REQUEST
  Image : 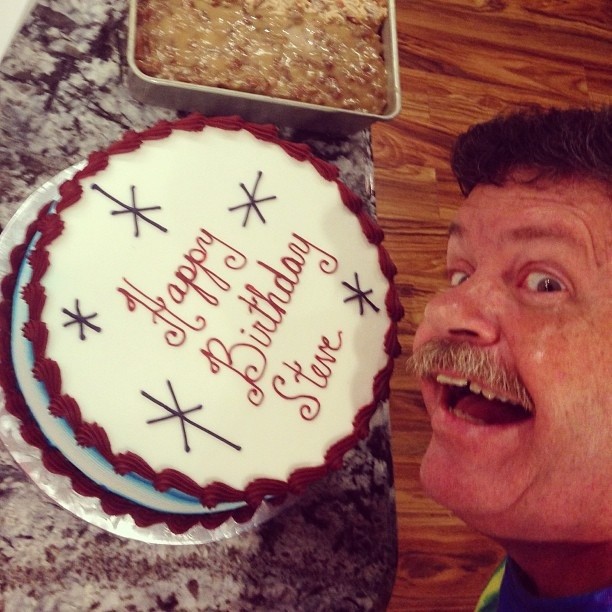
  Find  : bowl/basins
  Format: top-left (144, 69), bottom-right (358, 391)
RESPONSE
top-left (126, 0), bottom-right (403, 140)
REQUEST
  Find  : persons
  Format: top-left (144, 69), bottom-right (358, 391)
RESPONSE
top-left (410, 105), bottom-right (611, 608)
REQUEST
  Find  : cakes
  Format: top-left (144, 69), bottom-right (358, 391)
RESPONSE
top-left (0, 112), bottom-right (405, 547)
